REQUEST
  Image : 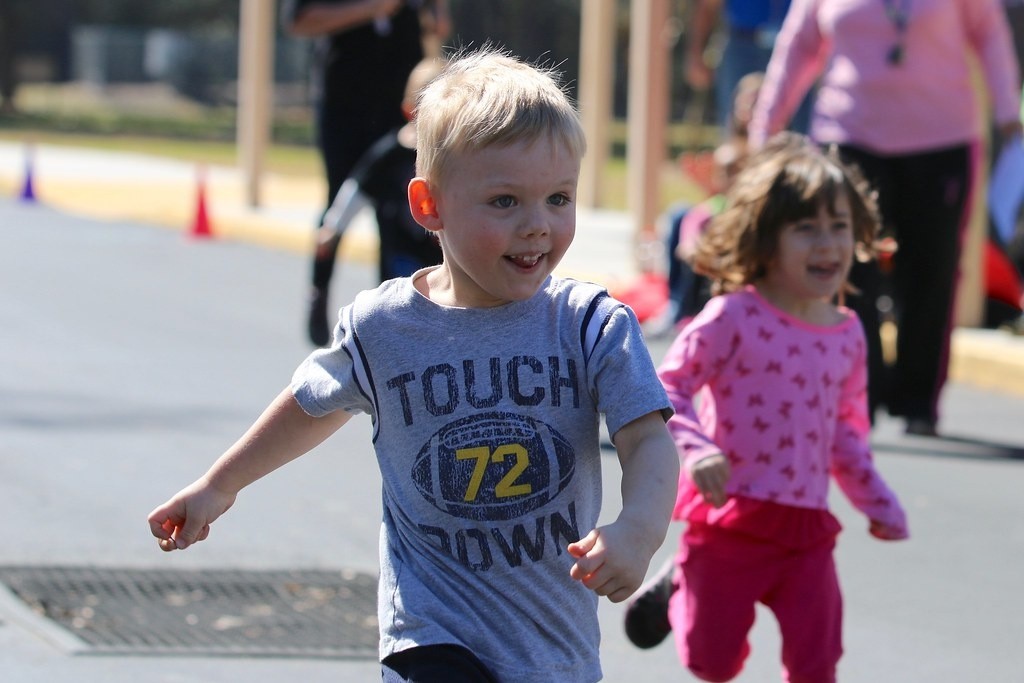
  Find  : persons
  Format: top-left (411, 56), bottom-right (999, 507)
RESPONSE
top-left (288, 1), bottom-right (1024, 683)
top-left (147, 49), bottom-right (680, 683)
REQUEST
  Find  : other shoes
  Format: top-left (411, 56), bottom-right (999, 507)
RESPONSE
top-left (641, 320), bottom-right (674, 339)
top-left (907, 421), bottom-right (936, 435)
top-left (310, 298), bottom-right (330, 344)
top-left (625, 560), bottom-right (680, 650)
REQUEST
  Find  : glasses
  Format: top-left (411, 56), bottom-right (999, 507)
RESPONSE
top-left (888, 9), bottom-right (909, 66)
